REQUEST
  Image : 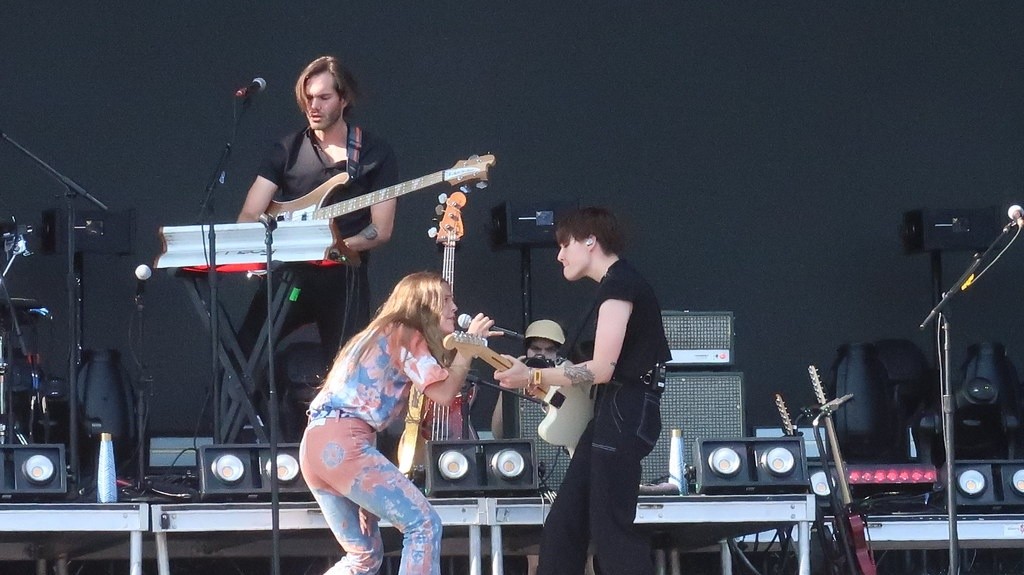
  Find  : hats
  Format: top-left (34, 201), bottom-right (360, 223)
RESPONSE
top-left (525, 320), bottom-right (566, 344)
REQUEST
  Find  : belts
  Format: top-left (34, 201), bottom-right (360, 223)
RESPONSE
top-left (311, 408), bottom-right (359, 419)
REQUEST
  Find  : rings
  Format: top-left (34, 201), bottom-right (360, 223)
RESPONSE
top-left (488, 331), bottom-right (491, 336)
top-left (482, 319), bottom-right (485, 324)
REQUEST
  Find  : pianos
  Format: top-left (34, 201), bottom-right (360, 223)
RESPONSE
top-left (152, 218), bottom-right (361, 443)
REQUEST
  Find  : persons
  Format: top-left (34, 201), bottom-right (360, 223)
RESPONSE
top-left (220, 56), bottom-right (400, 502)
top-left (491, 319), bottom-right (596, 575)
top-left (298, 273), bottom-right (504, 575)
top-left (492, 208), bottom-right (672, 575)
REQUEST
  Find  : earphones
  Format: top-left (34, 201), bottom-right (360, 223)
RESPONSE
top-left (586, 238), bottom-right (593, 246)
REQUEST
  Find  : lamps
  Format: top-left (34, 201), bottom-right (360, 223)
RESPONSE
top-left (76, 347), bottom-right (139, 473)
top-left (958, 342), bottom-right (1023, 459)
top-left (827, 338), bottom-right (901, 460)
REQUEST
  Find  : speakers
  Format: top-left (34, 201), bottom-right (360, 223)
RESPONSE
top-left (512, 371), bottom-right (746, 498)
top-left (662, 309), bottom-right (736, 369)
top-left (904, 203), bottom-right (1007, 255)
top-left (491, 198), bottom-right (581, 249)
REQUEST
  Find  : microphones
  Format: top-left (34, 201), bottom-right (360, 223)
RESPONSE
top-left (458, 314), bottom-right (529, 340)
top-left (236, 78), bottom-right (266, 96)
top-left (134, 264), bottom-right (152, 297)
top-left (1008, 204), bottom-right (1024, 228)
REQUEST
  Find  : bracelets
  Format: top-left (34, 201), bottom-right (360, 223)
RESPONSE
top-left (527, 366), bottom-right (541, 392)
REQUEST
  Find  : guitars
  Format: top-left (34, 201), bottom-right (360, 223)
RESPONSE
top-left (773, 391), bottom-right (844, 575)
top-left (396, 191), bottom-right (477, 479)
top-left (808, 364), bottom-right (877, 575)
top-left (246, 153), bottom-right (496, 276)
top-left (443, 332), bottom-right (596, 458)
